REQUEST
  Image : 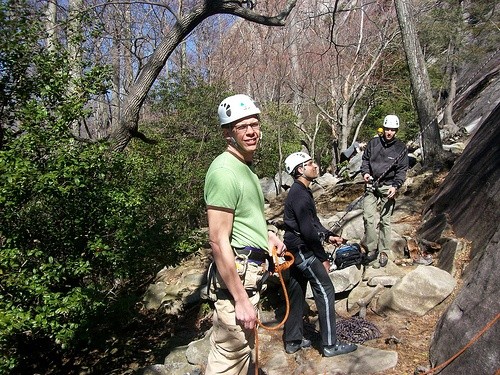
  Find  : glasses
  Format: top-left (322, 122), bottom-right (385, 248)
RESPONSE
top-left (232, 124), bottom-right (258, 133)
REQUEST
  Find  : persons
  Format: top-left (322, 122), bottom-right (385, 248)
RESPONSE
top-left (203, 94), bottom-right (287, 375)
top-left (374, 128), bottom-right (384, 138)
top-left (360, 115), bottom-right (409, 266)
top-left (283, 152), bottom-right (359, 356)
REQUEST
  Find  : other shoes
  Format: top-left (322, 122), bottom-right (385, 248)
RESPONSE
top-left (379, 252), bottom-right (388, 266)
top-left (323, 340), bottom-right (358, 356)
top-left (285, 339), bottom-right (311, 354)
top-left (367, 251), bottom-right (376, 260)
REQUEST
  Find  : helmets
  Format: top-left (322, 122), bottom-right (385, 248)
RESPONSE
top-left (284, 151), bottom-right (313, 173)
top-left (216, 94), bottom-right (261, 125)
top-left (378, 128), bottom-right (383, 133)
top-left (382, 114), bottom-right (399, 128)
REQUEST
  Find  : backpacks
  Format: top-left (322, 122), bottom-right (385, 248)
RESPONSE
top-left (334, 243), bottom-right (363, 270)
